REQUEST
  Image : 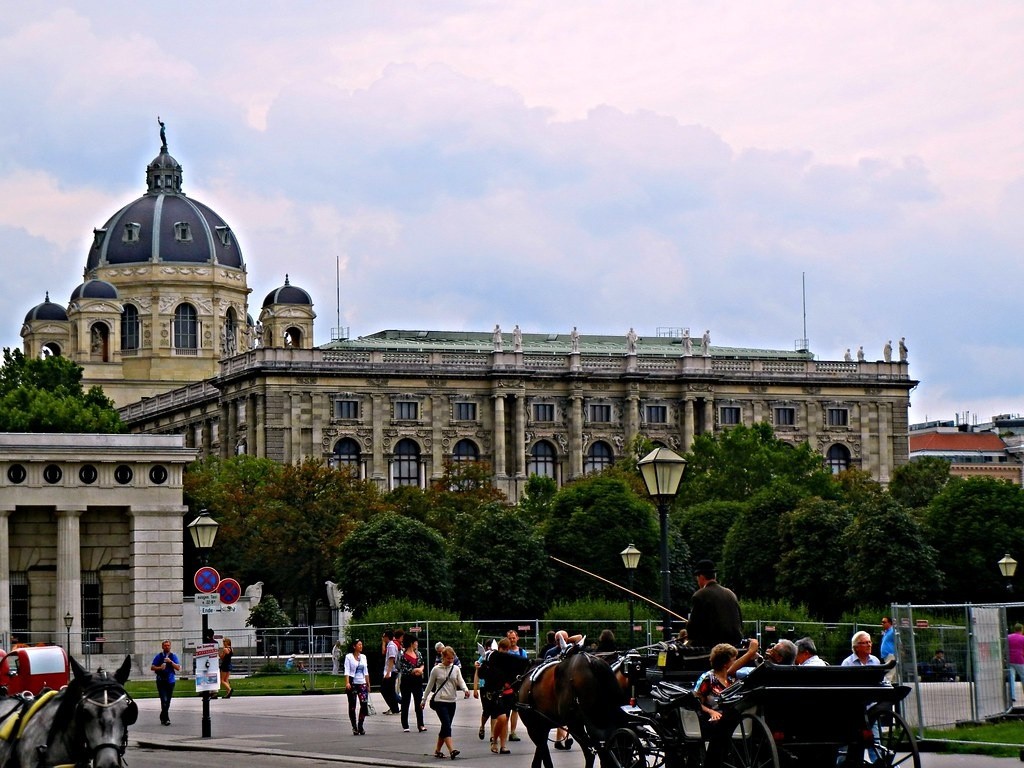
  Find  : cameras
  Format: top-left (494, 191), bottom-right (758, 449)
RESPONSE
top-left (741, 638), bottom-right (760, 648)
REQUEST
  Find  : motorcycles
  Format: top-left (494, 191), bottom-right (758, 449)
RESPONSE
top-left (0, 645), bottom-right (70, 696)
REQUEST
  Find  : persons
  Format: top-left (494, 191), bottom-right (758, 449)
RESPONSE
top-left (1007, 623), bottom-right (1024, 701)
top-left (626, 327), bottom-right (637, 354)
top-left (473, 630), bottom-right (618, 754)
top-left (702, 330), bottom-right (711, 355)
top-left (243, 321), bottom-right (264, 349)
top-left (380, 628), bottom-right (462, 733)
top-left (857, 346), bottom-right (864, 361)
top-left (682, 329), bottom-right (693, 355)
top-left (222, 330), bottom-right (234, 357)
top-left (513, 325), bottom-right (522, 351)
top-left (898, 337), bottom-right (908, 361)
top-left (493, 325), bottom-right (502, 350)
top-left (344, 639), bottom-right (371, 736)
top-left (844, 349), bottom-right (852, 361)
top-left (158, 117), bottom-right (166, 147)
top-left (284, 333), bottom-right (291, 348)
top-left (151, 640), bottom-right (181, 726)
top-left (678, 560), bottom-right (746, 645)
top-left (571, 327), bottom-right (579, 352)
top-left (92, 332), bottom-right (103, 354)
top-left (692, 614), bottom-right (905, 763)
top-left (883, 341), bottom-right (892, 362)
top-left (332, 640), bottom-right (342, 674)
top-left (286, 654), bottom-right (300, 670)
top-left (420, 646), bottom-right (470, 760)
top-left (207, 628), bottom-right (233, 699)
top-left (930, 649), bottom-right (956, 682)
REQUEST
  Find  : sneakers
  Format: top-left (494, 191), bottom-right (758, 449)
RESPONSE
top-left (383, 709), bottom-right (393, 715)
top-left (161, 719), bottom-right (170, 724)
top-left (164, 719), bottom-right (171, 726)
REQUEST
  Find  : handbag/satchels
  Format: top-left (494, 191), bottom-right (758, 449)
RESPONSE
top-left (430, 699), bottom-right (435, 710)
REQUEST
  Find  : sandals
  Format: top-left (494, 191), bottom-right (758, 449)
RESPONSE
top-left (450, 750), bottom-right (460, 760)
top-left (434, 750), bottom-right (446, 758)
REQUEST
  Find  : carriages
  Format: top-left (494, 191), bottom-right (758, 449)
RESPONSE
top-left (477, 627), bottom-right (919, 768)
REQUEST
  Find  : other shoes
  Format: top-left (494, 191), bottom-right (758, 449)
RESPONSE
top-left (500, 747), bottom-right (510, 755)
top-left (479, 727), bottom-right (485, 740)
top-left (353, 728), bottom-right (359, 736)
top-left (554, 741), bottom-right (566, 750)
top-left (359, 727), bottom-right (365, 735)
top-left (509, 733), bottom-right (521, 742)
top-left (404, 728), bottom-right (409, 732)
top-left (491, 742), bottom-right (498, 754)
top-left (566, 738), bottom-right (573, 750)
top-left (418, 726), bottom-right (427, 733)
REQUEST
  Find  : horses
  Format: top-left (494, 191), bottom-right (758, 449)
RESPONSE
top-left (0, 654), bottom-right (139, 768)
top-left (475, 641), bottom-right (630, 768)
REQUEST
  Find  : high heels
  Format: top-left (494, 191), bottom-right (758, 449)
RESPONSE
top-left (222, 688), bottom-right (234, 699)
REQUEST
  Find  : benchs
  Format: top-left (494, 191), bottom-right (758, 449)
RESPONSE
top-left (659, 646), bottom-right (755, 680)
top-left (728, 661), bottom-right (898, 732)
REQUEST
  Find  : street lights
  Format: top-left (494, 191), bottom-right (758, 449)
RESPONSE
top-left (997, 553), bottom-right (1018, 625)
top-left (636, 439), bottom-right (689, 648)
top-left (187, 507), bottom-right (222, 738)
top-left (63, 611), bottom-right (74, 656)
top-left (620, 541), bottom-right (643, 650)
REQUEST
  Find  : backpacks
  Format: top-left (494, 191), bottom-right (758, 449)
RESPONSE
top-left (395, 650), bottom-right (404, 671)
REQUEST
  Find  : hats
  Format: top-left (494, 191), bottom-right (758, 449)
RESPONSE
top-left (694, 560), bottom-right (720, 574)
